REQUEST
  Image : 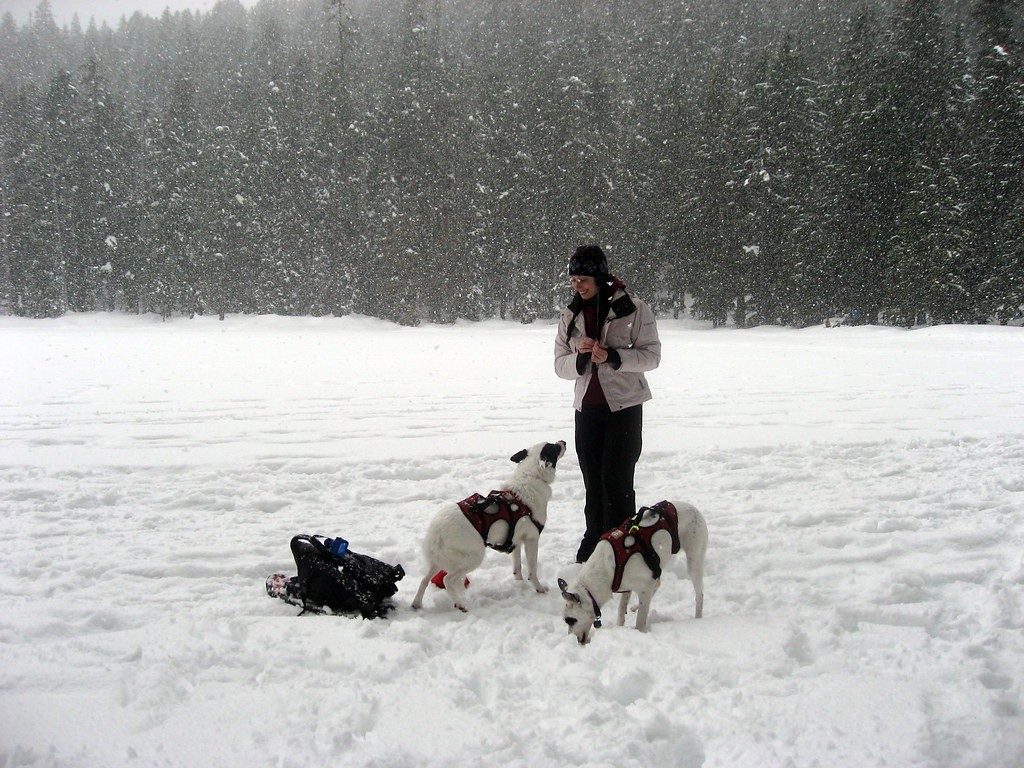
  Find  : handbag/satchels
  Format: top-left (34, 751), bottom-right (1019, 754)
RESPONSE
top-left (290, 535), bottom-right (406, 615)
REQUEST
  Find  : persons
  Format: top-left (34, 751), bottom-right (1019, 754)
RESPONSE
top-left (555, 245), bottom-right (661, 564)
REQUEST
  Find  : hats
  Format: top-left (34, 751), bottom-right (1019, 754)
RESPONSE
top-left (569, 245), bottom-right (608, 288)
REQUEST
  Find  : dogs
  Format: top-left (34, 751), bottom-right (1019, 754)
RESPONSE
top-left (411, 440), bottom-right (567, 613)
top-left (556, 501), bottom-right (709, 645)
